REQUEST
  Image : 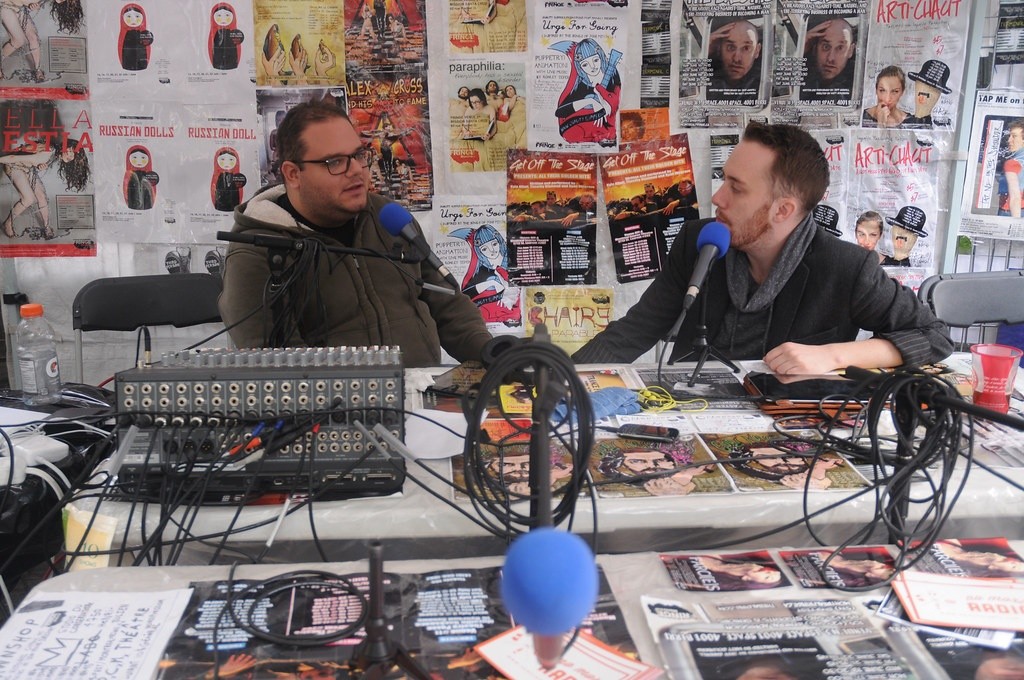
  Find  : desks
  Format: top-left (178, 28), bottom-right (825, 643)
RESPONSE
top-left (2, 363), bottom-right (1024, 680)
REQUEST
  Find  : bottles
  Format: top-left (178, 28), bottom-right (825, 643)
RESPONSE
top-left (15, 304), bottom-right (62, 407)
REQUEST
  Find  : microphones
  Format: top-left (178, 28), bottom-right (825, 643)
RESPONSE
top-left (682, 221), bottom-right (731, 311)
top-left (498, 323), bottom-right (601, 636)
top-left (377, 203), bottom-right (458, 289)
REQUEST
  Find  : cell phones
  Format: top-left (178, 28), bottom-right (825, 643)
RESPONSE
top-left (617, 423), bottom-right (679, 443)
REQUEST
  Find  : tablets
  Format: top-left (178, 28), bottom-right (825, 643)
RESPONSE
top-left (747, 372), bottom-right (893, 406)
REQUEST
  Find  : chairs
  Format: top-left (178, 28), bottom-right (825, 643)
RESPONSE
top-left (72, 274), bottom-right (234, 384)
top-left (917, 269), bottom-right (1024, 330)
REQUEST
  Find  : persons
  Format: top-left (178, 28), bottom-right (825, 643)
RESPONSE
top-left (180, 647), bottom-right (509, 680)
top-left (947, 647), bottom-right (1024, 680)
top-left (508, 190), bottom-right (596, 226)
top-left (799, 17), bottom-right (856, 107)
top-left (360, 123), bottom-right (414, 193)
top-left (997, 119), bottom-right (1024, 219)
top-left (714, 655), bottom-right (806, 680)
top-left (344, 0), bottom-right (409, 65)
top-left (0, 0), bottom-right (84, 80)
top-left (606, 179), bottom-right (696, 221)
top-left (811, 551), bottom-right (898, 588)
top-left (219, 95), bottom-right (493, 369)
top-left (706, 19), bottom-right (762, 107)
top-left (855, 211), bottom-right (892, 265)
top-left (698, 554), bottom-right (784, 592)
top-left (569, 121), bottom-right (955, 375)
top-left (449, 80), bottom-right (527, 173)
top-left (449, 0), bottom-right (528, 54)
top-left (621, 113), bottom-right (644, 142)
top-left (935, 538), bottom-right (1024, 579)
top-left (0, 139), bottom-right (91, 238)
top-left (861, 65), bottom-right (912, 129)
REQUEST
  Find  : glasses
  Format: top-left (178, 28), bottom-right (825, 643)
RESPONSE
top-left (577, 200), bottom-right (593, 207)
top-left (292, 145), bottom-right (375, 175)
top-left (469, 100), bottom-right (481, 105)
top-left (677, 185), bottom-right (694, 192)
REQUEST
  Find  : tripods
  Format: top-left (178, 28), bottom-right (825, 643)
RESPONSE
top-left (666, 257), bottom-right (740, 388)
top-left (348, 544), bottom-right (433, 680)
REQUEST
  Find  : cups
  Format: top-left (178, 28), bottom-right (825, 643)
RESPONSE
top-left (969, 344), bottom-right (1024, 415)
